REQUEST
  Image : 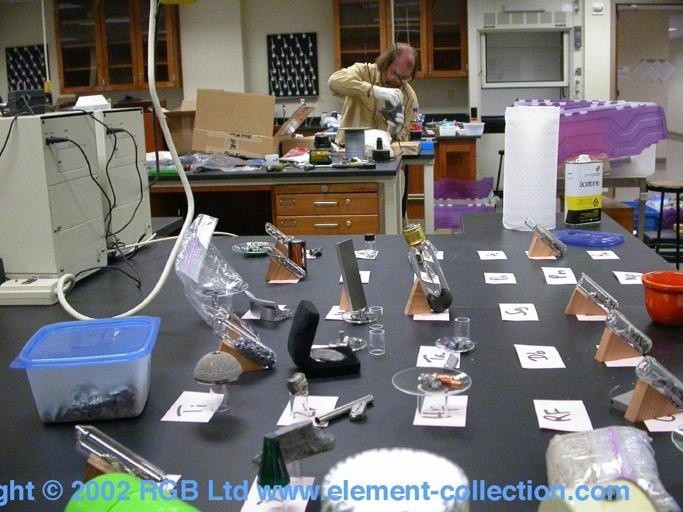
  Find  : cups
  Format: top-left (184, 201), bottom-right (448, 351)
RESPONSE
top-left (453, 317), bottom-right (470, 342)
top-left (365, 305), bottom-right (386, 356)
top-left (372, 149), bottom-right (390, 162)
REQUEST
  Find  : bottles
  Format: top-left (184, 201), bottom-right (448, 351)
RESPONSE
top-left (402, 220), bottom-right (453, 314)
top-left (74, 423), bottom-right (164, 484)
top-left (267, 248), bottom-right (307, 279)
top-left (409, 106), bottom-right (423, 141)
top-left (364, 232), bottom-right (376, 258)
top-left (523, 216), bottom-right (683, 457)
top-left (211, 321), bottom-right (277, 370)
top-left (256, 433), bottom-right (290, 488)
top-left (264, 222), bottom-right (290, 248)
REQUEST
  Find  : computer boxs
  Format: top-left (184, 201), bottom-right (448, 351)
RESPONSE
top-left (2, 113), bottom-right (109, 287)
top-left (95, 105), bottom-right (152, 259)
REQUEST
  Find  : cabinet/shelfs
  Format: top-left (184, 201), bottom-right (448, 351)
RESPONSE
top-left (558, 176), bottom-right (646, 242)
top-left (111, 99), bottom-right (167, 151)
top-left (407, 138), bottom-right (476, 221)
top-left (332, 0), bottom-right (468, 79)
top-left (51, 0), bottom-right (182, 94)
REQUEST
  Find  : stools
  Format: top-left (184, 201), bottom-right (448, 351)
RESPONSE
top-left (643, 178), bottom-right (683, 271)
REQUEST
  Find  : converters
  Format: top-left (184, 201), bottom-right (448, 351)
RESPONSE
top-left (9, 89), bottom-right (46, 114)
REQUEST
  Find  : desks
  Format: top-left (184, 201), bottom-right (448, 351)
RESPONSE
top-left (559, 192), bottom-right (633, 235)
top-left (0, 215), bottom-right (683, 512)
top-left (146, 151), bottom-right (404, 234)
top-left (400, 157), bottom-right (434, 233)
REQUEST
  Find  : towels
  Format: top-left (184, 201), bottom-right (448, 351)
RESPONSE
top-left (516, 96), bottom-right (669, 159)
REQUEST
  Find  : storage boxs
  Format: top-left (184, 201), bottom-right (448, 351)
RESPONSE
top-left (191, 88), bottom-right (315, 159)
top-left (11, 315), bottom-right (163, 422)
top-left (147, 104), bottom-right (195, 156)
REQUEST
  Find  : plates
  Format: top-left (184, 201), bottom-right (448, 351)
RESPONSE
top-left (340, 310), bottom-right (376, 324)
top-left (231, 242), bottom-right (271, 255)
top-left (327, 337), bottom-right (368, 352)
top-left (391, 366), bottom-right (473, 398)
top-left (434, 336), bottom-right (475, 353)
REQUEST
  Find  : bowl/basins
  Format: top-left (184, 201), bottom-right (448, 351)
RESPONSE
top-left (455, 121), bottom-right (486, 136)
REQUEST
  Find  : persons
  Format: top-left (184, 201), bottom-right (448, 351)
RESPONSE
top-left (328, 43), bottom-right (419, 230)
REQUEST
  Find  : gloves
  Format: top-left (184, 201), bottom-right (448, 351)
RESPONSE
top-left (385, 112), bottom-right (405, 136)
top-left (369, 85), bottom-right (404, 109)
